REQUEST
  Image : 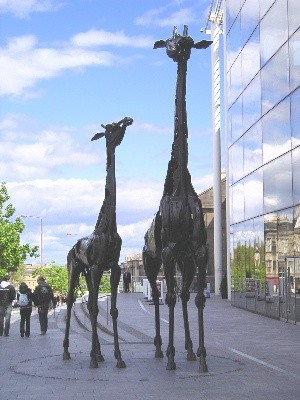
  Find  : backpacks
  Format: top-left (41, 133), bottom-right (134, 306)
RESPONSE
top-left (18, 290), bottom-right (30, 306)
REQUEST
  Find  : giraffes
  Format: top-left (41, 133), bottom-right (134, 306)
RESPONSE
top-left (62, 117), bottom-right (134, 369)
top-left (142, 24), bottom-right (214, 373)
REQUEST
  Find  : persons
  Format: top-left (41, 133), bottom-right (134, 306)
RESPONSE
top-left (123, 268), bottom-right (132, 293)
top-left (0, 274), bottom-right (16, 337)
top-left (16, 282), bottom-right (33, 338)
top-left (33, 276), bottom-right (57, 335)
